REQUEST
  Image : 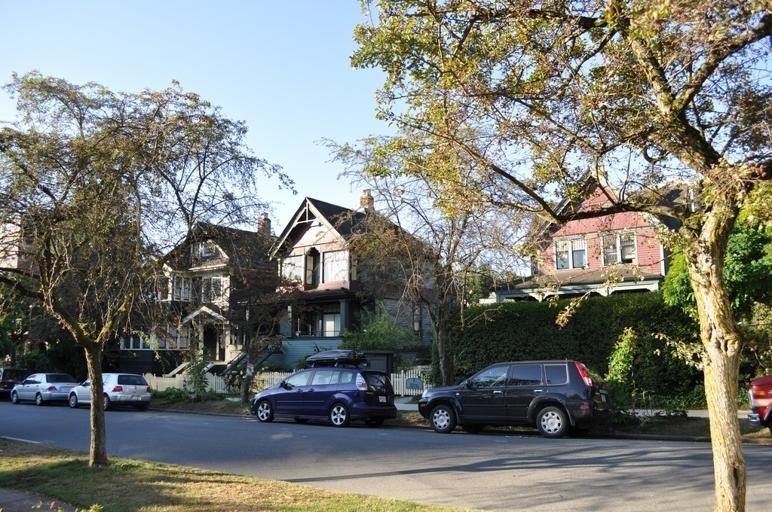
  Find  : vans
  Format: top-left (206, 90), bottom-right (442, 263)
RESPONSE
top-left (249, 349), bottom-right (397, 428)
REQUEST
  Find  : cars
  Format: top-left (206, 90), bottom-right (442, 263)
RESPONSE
top-left (418, 358), bottom-right (612, 438)
top-left (747, 374), bottom-right (772, 436)
top-left (0, 367), bottom-right (153, 411)
